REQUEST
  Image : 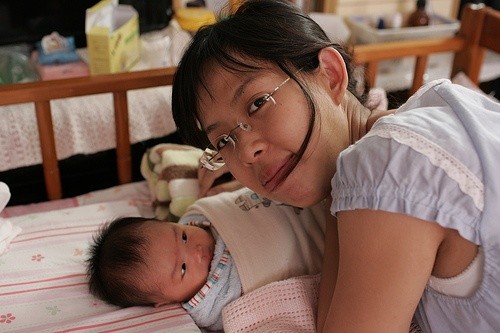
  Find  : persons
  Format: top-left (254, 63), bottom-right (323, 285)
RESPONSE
top-left (172, 0), bottom-right (500, 333)
top-left (87, 186), bottom-right (326, 331)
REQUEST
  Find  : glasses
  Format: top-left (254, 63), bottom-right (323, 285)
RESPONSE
top-left (199, 68), bottom-right (304, 170)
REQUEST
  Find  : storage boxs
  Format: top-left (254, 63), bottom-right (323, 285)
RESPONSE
top-left (85, 0), bottom-right (141, 75)
top-left (348, 12), bottom-right (461, 74)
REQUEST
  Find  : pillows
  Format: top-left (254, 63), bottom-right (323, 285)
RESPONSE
top-left (0, 198), bottom-right (202, 333)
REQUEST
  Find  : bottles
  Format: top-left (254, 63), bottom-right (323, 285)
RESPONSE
top-left (406, 0), bottom-right (429, 27)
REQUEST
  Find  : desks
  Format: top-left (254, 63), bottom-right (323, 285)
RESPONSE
top-left (0, 13), bottom-right (350, 207)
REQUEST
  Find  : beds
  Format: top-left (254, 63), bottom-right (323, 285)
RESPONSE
top-left (0, 4), bottom-right (500, 333)
